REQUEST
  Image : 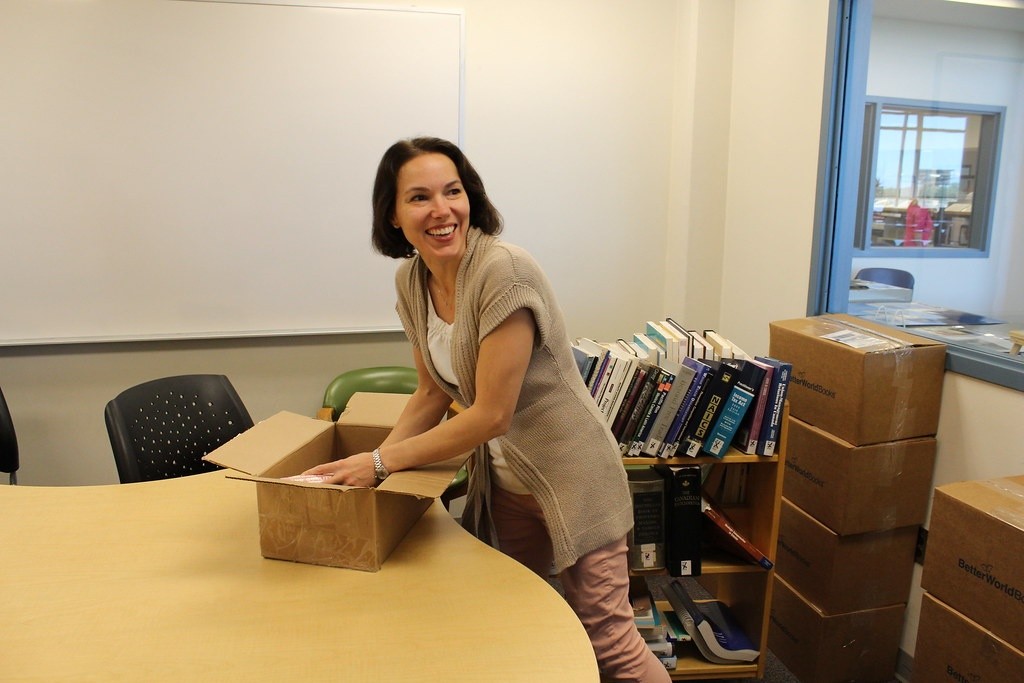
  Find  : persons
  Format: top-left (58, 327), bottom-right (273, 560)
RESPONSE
top-left (904, 185), bottom-right (934, 247)
top-left (300, 138), bottom-right (677, 683)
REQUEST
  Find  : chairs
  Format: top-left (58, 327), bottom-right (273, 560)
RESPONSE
top-left (854, 268), bottom-right (915, 290)
top-left (316, 367), bottom-right (470, 513)
top-left (104, 373), bottom-right (255, 485)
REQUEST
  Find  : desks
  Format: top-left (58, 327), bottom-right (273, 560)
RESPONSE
top-left (0, 469), bottom-right (602, 683)
top-left (848, 279), bottom-right (1024, 359)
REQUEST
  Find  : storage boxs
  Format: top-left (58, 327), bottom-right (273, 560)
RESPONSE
top-left (766, 572), bottom-right (907, 683)
top-left (769, 313), bottom-right (949, 447)
top-left (921, 475), bottom-right (1024, 653)
top-left (201, 391), bottom-right (475, 573)
top-left (781, 414), bottom-right (937, 537)
top-left (774, 496), bottom-right (917, 616)
top-left (910, 590), bottom-right (1024, 683)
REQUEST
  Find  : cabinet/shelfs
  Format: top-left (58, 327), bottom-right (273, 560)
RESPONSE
top-left (621, 400), bottom-right (790, 683)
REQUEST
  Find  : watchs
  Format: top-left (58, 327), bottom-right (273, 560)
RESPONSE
top-left (371, 449), bottom-right (389, 480)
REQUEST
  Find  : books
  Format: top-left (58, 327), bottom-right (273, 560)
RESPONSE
top-left (616, 574), bottom-right (760, 670)
top-left (623, 460), bottom-right (774, 576)
top-left (567, 319), bottom-right (793, 461)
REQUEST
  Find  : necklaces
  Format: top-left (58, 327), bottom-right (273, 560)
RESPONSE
top-left (436, 282), bottom-right (455, 314)
